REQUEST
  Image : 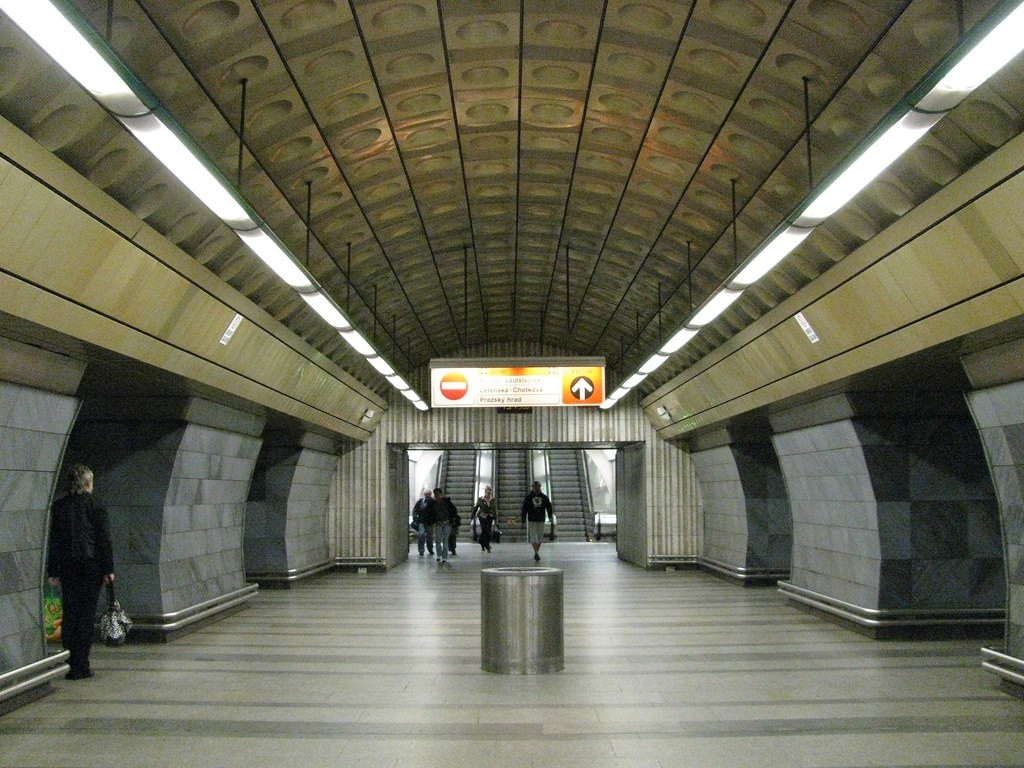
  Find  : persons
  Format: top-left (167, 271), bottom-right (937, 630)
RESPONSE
top-left (431, 488), bottom-right (458, 562)
top-left (48, 463), bottom-right (114, 680)
top-left (521, 481), bottom-right (553, 561)
top-left (413, 488), bottom-right (436, 556)
top-left (470, 484), bottom-right (499, 553)
top-left (448, 515), bottom-right (461, 555)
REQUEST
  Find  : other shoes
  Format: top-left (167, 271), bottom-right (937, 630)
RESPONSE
top-left (482, 546), bottom-right (485, 551)
top-left (65, 660), bottom-right (95, 679)
top-left (419, 553), bottom-right (423, 557)
top-left (534, 553), bottom-right (540, 561)
top-left (429, 551), bottom-right (434, 554)
top-left (437, 556), bottom-right (440, 561)
top-left (451, 550), bottom-right (456, 555)
top-left (487, 549), bottom-right (491, 553)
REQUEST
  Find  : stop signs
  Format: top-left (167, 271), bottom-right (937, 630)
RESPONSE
top-left (440, 372), bottom-right (468, 400)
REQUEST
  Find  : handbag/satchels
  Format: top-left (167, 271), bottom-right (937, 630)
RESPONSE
top-left (411, 520), bottom-right (419, 531)
top-left (102, 576), bottom-right (133, 647)
top-left (44, 582), bottom-right (62, 640)
top-left (493, 530), bottom-right (500, 543)
